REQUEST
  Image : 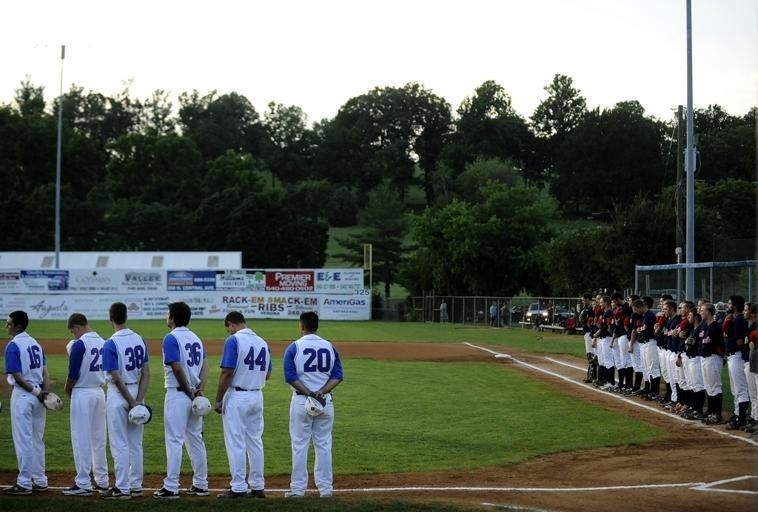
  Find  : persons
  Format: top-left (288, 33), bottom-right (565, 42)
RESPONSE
top-left (214, 310), bottom-right (272, 498)
top-left (439, 298), bottom-right (580, 334)
top-left (152, 300), bottom-right (211, 496)
top-left (580, 289), bottom-right (758, 436)
top-left (101, 303), bottom-right (150, 499)
top-left (283, 311), bottom-right (344, 498)
top-left (2, 311), bottom-right (49, 497)
top-left (59, 312), bottom-right (111, 498)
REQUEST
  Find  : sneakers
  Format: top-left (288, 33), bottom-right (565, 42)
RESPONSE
top-left (724, 415), bottom-right (757, 438)
top-left (284, 492), bottom-right (302, 498)
top-left (185, 485), bottom-right (209, 496)
top-left (217, 489), bottom-right (247, 499)
top-left (583, 377), bottom-right (617, 391)
top-left (250, 489), bottom-right (266, 499)
top-left (62, 483), bottom-right (143, 500)
top-left (153, 487), bottom-right (179, 500)
top-left (657, 398), bottom-right (722, 425)
top-left (31, 481), bottom-right (48, 492)
top-left (2, 484), bottom-right (32, 495)
top-left (610, 386), bottom-right (661, 401)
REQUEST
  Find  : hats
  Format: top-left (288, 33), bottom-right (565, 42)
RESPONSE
top-left (306, 391), bottom-right (326, 417)
top-left (192, 396), bottom-right (211, 417)
top-left (44, 392), bottom-right (63, 411)
top-left (128, 403), bottom-right (152, 426)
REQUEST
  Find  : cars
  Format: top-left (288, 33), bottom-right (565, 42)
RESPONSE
top-left (551, 305), bottom-right (574, 324)
top-left (47, 275), bottom-right (66, 291)
top-left (511, 305), bottom-right (523, 320)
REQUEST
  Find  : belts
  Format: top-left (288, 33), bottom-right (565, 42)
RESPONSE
top-left (236, 386), bottom-right (247, 391)
top-left (296, 391), bottom-right (304, 395)
top-left (176, 387), bottom-right (184, 392)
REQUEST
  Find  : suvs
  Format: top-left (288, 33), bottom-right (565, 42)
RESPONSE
top-left (524, 303), bottom-right (550, 325)
